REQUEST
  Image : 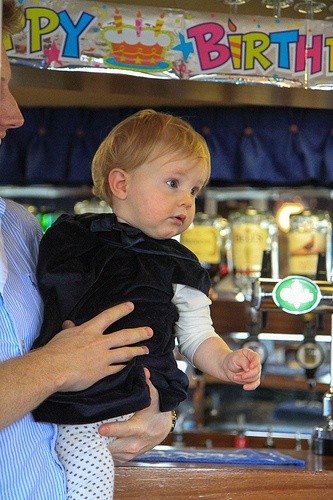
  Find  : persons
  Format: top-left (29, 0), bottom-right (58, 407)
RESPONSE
top-left (1, 1), bottom-right (176, 499)
top-left (28, 110), bottom-right (263, 499)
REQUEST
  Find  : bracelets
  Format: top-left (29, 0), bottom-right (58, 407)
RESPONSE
top-left (171, 410), bottom-right (176, 432)
